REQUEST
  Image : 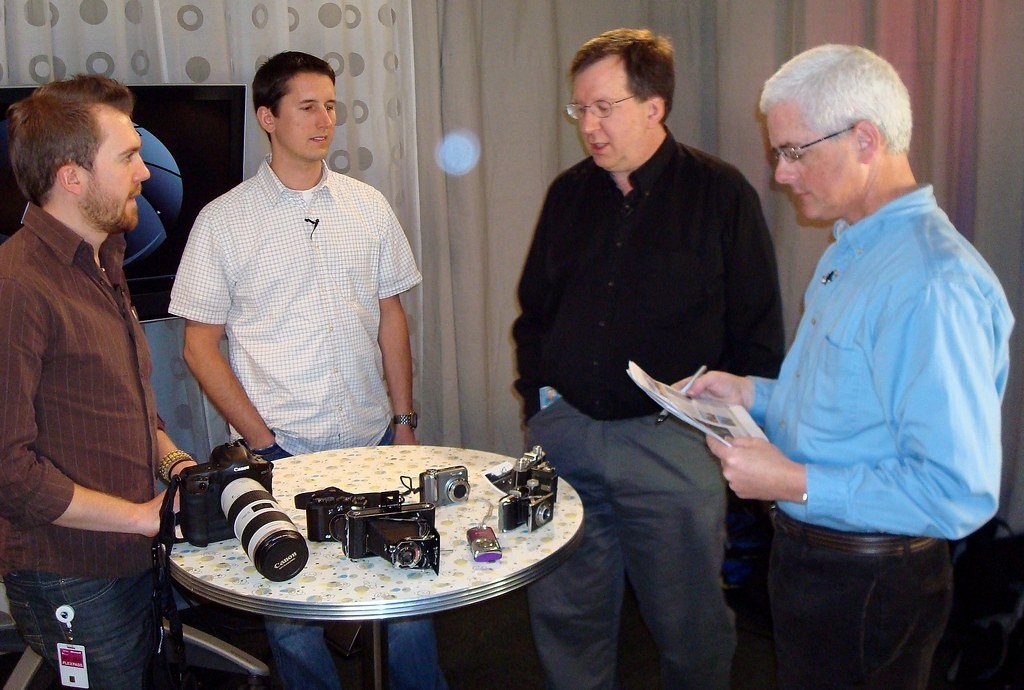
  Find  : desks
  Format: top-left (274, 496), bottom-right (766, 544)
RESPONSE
top-left (170, 445), bottom-right (585, 690)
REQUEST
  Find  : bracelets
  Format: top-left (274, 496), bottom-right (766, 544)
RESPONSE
top-left (157, 449), bottom-right (193, 482)
top-left (799, 492), bottom-right (808, 506)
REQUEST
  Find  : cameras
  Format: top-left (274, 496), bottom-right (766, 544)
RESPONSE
top-left (498, 488), bottom-right (555, 533)
top-left (305, 490), bottom-right (399, 543)
top-left (419, 466), bottom-right (471, 507)
top-left (344, 502), bottom-right (438, 570)
top-left (175, 441), bottom-right (310, 582)
top-left (513, 444), bottom-right (559, 500)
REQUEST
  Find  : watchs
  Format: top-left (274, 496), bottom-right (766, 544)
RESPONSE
top-left (392, 412), bottom-right (417, 429)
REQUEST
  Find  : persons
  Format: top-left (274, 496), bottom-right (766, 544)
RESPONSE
top-left (166, 51), bottom-right (450, 690)
top-left (0, 74), bottom-right (199, 690)
top-left (511, 27), bottom-right (786, 690)
top-left (671, 43), bottom-right (1016, 690)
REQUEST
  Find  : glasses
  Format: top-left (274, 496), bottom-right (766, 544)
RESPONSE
top-left (770, 124), bottom-right (856, 165)
top-left (566, 93), bottom-right (640, 120)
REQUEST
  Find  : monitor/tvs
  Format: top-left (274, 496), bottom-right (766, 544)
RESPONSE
top-left (1, 84), bottom-right (247, 324)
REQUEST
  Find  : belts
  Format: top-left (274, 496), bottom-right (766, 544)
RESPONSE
top-left (769, 502), bottom-right (938, 556)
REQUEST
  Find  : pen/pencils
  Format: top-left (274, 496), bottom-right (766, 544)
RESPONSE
top-left (656, 364), bottom-right (706, 424)
